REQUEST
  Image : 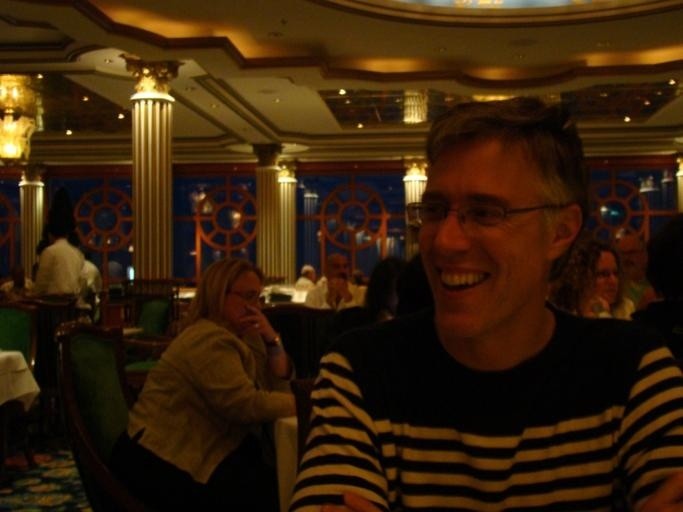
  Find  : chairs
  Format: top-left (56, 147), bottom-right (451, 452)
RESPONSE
top-left (0, 277), bottom-right (336, 511)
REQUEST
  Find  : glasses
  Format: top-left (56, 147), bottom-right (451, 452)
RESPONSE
top-left (227, 292), bottom-right (265, 306)
top-left (408, 202), bottom-right (557, 229)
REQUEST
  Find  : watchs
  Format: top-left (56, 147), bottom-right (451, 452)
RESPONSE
top-left (264, 331), bottom-right (280, 348)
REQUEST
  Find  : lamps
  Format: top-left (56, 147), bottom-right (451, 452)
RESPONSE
top-left (0, 72), bottom-right (40, 167)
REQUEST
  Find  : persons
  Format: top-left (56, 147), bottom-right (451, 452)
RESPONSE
top-left (125, 257), bottom-right (297, 512)
top-left (1, 218), bottom-right (104, 385)
top-left (550, 213), bottom-right (683, 369)
top-left (287, 104), bottom-right (683, 511)
top-left (289, 251), bottom-right (436, 380)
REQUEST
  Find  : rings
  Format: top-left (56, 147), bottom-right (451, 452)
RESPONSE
top-left (255, 323), bottom-right (261, 329)
